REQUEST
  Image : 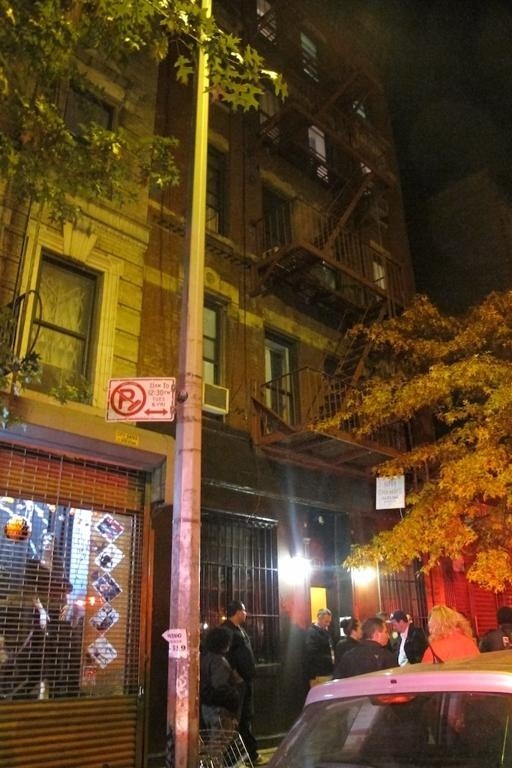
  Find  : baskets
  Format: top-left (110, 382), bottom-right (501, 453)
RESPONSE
top-left (199, 729), bottom-right (254, 768)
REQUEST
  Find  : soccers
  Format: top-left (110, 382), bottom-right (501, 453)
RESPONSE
top-left (4, 515), bottom-right (33, 541)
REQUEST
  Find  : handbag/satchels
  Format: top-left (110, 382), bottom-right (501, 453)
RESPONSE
top-left (201, 675), bottom-right (242, 712)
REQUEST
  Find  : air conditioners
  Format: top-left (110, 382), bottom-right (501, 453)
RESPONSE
top-left (203, 382), bottom-right (230, 417)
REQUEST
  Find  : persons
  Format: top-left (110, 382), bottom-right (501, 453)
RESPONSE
top-left (219, 600), bottom-right (271, 766)
top-left (304, 603), bottom-right (512, 733)
top-left (199, 627), bottom-right (244, 757)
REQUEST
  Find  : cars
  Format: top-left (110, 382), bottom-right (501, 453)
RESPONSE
top-left (269, 647), bottom-right (512, 768)
